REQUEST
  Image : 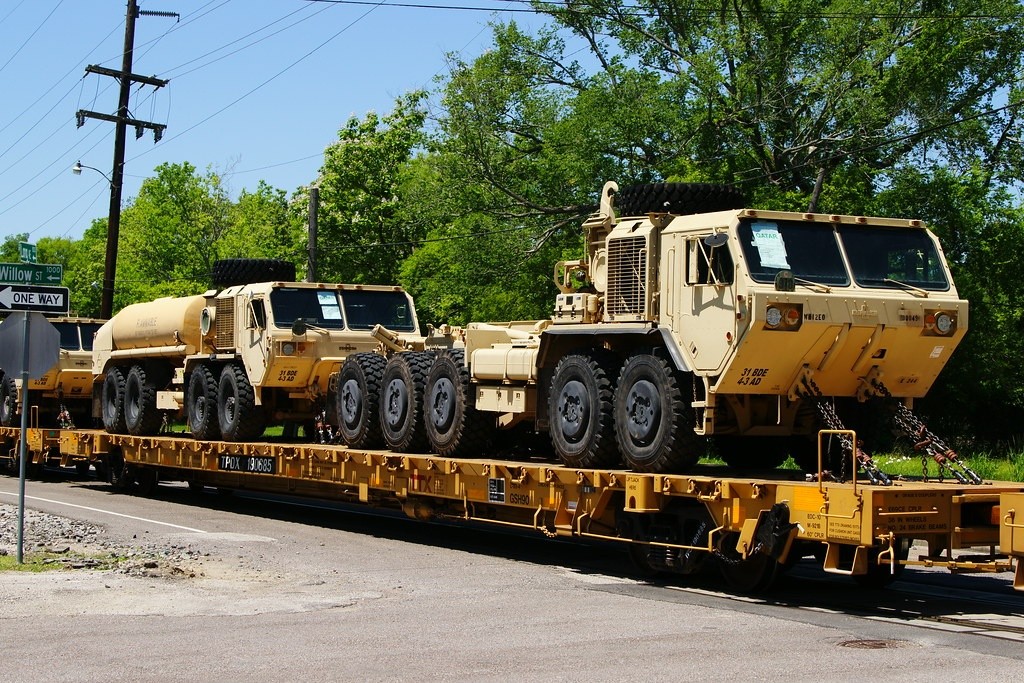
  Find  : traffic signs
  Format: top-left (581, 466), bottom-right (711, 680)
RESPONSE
top-left (2, 286), bottom-right (68, 308)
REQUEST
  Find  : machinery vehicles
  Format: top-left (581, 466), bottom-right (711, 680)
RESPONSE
top-left (333, 183), bottom-right (971, 468)
top-left (0, 281), bottom-right (426, 441)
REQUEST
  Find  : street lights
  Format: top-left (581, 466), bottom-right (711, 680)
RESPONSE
top-left (74, 157), bottom-right (125, 320)
